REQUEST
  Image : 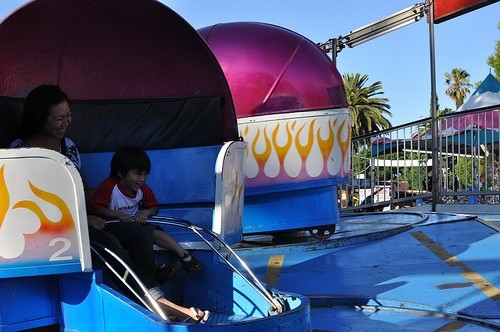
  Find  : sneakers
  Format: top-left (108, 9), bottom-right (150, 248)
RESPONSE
top-left (181, 253), bottom-right (203, 273)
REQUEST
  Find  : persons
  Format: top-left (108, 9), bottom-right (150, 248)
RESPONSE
top-left (8, 83), bottom-right (211, 325)
top-left (87, 145), bottom-right (204, 289)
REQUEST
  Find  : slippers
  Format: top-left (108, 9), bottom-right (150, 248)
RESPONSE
top-left (182, 307), bottom-right (210, 324)
